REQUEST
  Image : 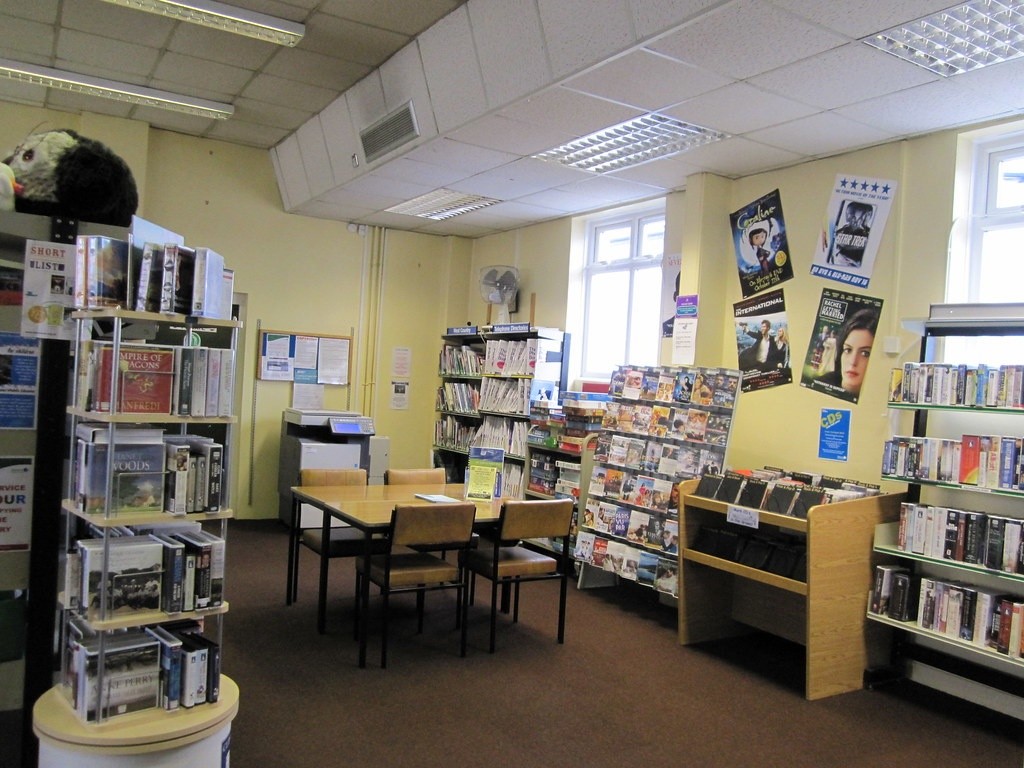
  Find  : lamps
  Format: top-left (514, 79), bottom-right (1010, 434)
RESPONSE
top-left (0, 58), bottom-right (236, 122)
top-left (104, 0), bottom-right (307, 48)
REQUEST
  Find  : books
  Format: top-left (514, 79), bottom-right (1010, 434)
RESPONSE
top-left (826, 200), bottom-right (879, 268)
top-left (435, 340), bottom-right (1024, 667)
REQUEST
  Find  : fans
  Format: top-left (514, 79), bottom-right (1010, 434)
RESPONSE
top-left (479, 263), bottom-right (520, 314)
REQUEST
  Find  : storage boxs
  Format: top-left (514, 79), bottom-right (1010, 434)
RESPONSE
top-left (526, 388), bottom-right (613, 452)
top-left (529, 512), bottom-right (577, 555)
top-left (58, 234), bottom-right (235, 723)
top-left (527, 453), bottom-right (582, 505)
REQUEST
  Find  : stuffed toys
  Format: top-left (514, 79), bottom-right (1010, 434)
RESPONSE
top-left (0, 125), bottom-right (138, 228)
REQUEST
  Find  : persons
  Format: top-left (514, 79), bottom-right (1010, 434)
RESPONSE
top-left (603, 554), bottom-right (614, 572)
top-left (625, 560), bottom-right (637, 573)
top-left (621, 475), bottom-right (634, 501)
top-left (833, 202), bottom-right (874, 265)
top-left (667, 568), bottom-right (679, 595)
top-left (739, 308), bottom-right (878, 396)
top-left (715, 375), bottom-right (724, 389)
top-left (657, 567), bottom-right (673, 593)
top-left (688, 411), bottom-right (698, 428)
top-left (668, 483), bottom-right (681, 508)
top-left (639, 483), bottom-right (646, 501)
top-left (727, 376), bottom-right (737, 392)
top-left (697, 413), bottom-right (706, 427)
top-left (632, 412), bottom-right (643, 426)
top-left (91, 565), bottom-right (161, 614)
top-left (661, 531), bottom-right (670, 550)
top-left (584, 508), bottom-right (594, 525)
top-left (693, 375), bottom-right (704, 403)
top-left (592, 473), bottom-right (606, 491)
top-left (667, 535), bottom-right (678, 553)
top-left (673, 420), bottom-right (686, 435)
top-left (651, 494), bottom-right (661, 508)
top-left (606, 403), bottom-right (613, 413)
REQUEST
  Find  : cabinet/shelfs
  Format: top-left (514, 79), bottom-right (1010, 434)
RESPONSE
top-left (861, 301), bottom-right (1024, 744)
top-left (0, 208), bottom-right (56, 714)
top-left (523, 423), bottom-right (598, 578)
top-left (575, 364), bottom-right (743, 609)
top-left (675, 473), bottom-right (910, 702)
top-left (433, 326), bottom-right (572, 482)
top-left (30, 304), bottom-right (241, 768)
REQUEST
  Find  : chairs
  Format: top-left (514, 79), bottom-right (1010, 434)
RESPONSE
top-left (292, 468), bottom-right (369, 604)
top-left (352, 501), bottom-right (477, 672)
top-left (455, 497), bottom-right (575, 657)
top-left (384, 468), bottom-right (448, 586)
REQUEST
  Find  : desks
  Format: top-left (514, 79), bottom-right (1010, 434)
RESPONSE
top-left (291, 482), bottom-right (526, 669)
